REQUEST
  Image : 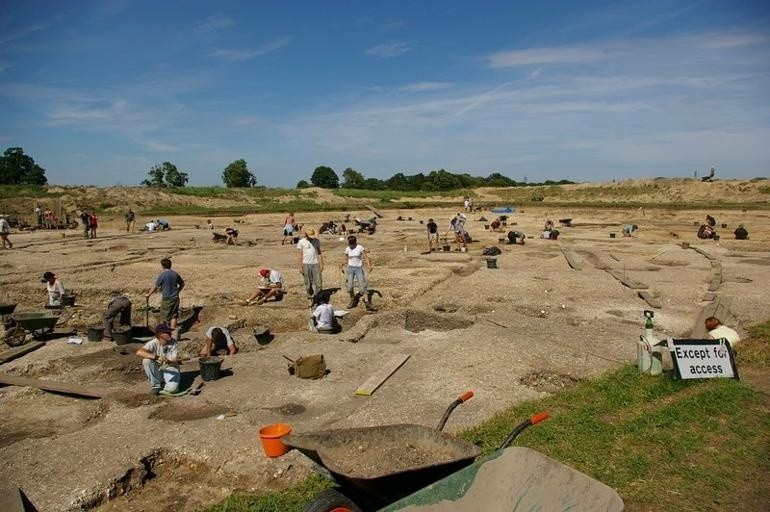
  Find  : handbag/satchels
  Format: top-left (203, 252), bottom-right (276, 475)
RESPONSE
top-left (295, 353), bottom-right (328, 379)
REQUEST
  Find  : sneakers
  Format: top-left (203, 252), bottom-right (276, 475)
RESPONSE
top-left (256, 299), bottom-right (263, 305)
top-left (366, 303), bottom-right (378, 312)
top-left (151, 389), bottom-right (162, 394)
top-left (237, 298), bottom-right (248, 306)
top-left (347, 297), bottom-right (356, 309)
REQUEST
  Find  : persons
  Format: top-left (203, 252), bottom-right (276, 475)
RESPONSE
top-left (697, 214), bottom-right (715, 239)
top-left (308, 290), bottom-right (335, 334)
top-left (297, 228), bottom-right (324, 298)
top-left (238, 269), bottom-right (285, 306)
top-left (206, 220), bottom-right (214, 230)
top-left (734, 223), bottom-right (748, 240)
top-left (100, 297), bottom-right (134, 343)
top-left (425, 198), bottom-right (474, 255)
top-left (143, 259), bottom-right (187, 327)
top-left (491, 216), bottom-right (509, 232)
top-left (80, 208), bottom-right (97, 239)
top-left (705, 317), bottom-right (741, 348)
top-left (35, 205), bottom-right (58, 229)
top-left (125, 208), bottom-right (136, 232)
top-left (0, 214), bottom-right (12, 249)
top-left (40, 271), bottom-right (65, 307)
top-left (508, 231), bottom-right (524, 244)
top-left (344, 236), bottom-right (373, 311)
top-left (143, 217), bottom-right (169, 233)
top-left (140, 323), bottom-right (185, 395)
top-left (282, 213), bottom-right (304, 245)
top-left (318, 215), bottom-right (377, 235)
top-left (224, 226), bottom-right (238, 246)
top-left (623, 224), bottom-right (637, 237)
top-left (201, 326), bottom-right (236, 359)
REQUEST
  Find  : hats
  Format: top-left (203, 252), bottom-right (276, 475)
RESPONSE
top-left (259, 270), bottom-right (267, 275)
top-left (304, 228), bottom-right (317, 239)
top-left (156, 324), bottom-right (175, 333)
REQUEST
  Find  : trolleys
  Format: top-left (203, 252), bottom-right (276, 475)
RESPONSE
top-left (0, 303), bottom-right (78, 347)
